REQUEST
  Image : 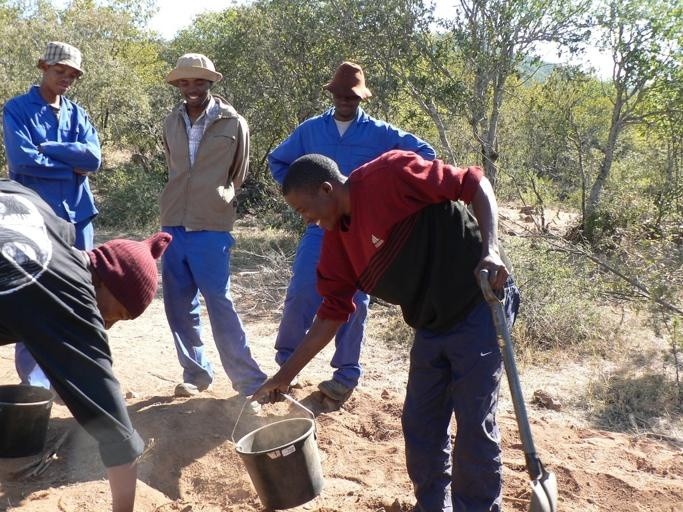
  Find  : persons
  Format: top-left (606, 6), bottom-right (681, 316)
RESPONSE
top-left (265, 60), bottom-right (437, 403)
top-left (0, 39), bottom-right (105, 407)
top-left (240, 151), bottom-right (524, 511)
top-left (0, 176), bottom-right (174, 512)
top-left (155, 52), bottom-right (269, 399)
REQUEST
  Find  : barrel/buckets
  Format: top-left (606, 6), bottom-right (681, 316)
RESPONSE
top-left (230, 394), bottom-right (325, 511)
top-left (1, 383), bottom-right (60, 460)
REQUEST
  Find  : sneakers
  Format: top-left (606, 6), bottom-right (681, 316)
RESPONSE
top-left (319, 379), bottom-right (353, 402)
top-left (175, 382), bottom-right (213, 396)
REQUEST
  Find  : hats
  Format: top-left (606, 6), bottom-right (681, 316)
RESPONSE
top-left (37, 42), bottom-right (84, 76)
top-left (166, 53), bottom-right (222, 89)
top-left (322, 63), bottom-right (372, 99)
top-left (88, 231), bottom-right (174, 318)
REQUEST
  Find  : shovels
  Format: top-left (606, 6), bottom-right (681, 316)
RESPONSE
top-left (479, 270), bottom-right (558, 512)
